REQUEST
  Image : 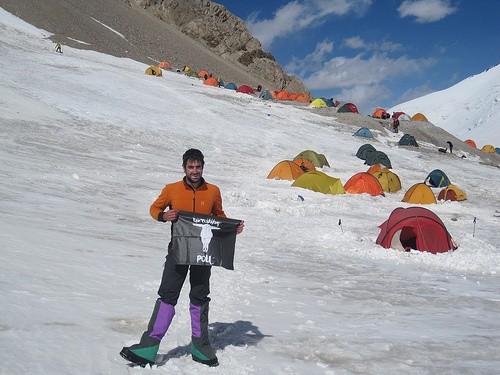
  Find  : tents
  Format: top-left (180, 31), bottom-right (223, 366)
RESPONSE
top-left (236, 85), bottom-right (311, 103)
top-left (266, 150), bottom-right (347, 194)
top-left (373, 109), bottom-right (428, 122)
top-left (464, 139), bottom-right (500, 155)
top-left (353, 127), bottom-right (373, 138)
top-left (308, 98), bottom-right (358, 113)
top-left (145, 66), bottom-right (162, 76)
top-left (399, 169), bottom-right (467, 204)
top-left (159, 61), bottom-right (171, 69)
top-left (377, 207), bottom-right (458, 254)
top-left (343, 143), bottom-right (402, 198)
top-left (398, 133), bottom-right (418, 147)
top-left (183, 65), bottom-right (237, 90)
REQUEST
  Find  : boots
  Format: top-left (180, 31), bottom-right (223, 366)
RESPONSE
top-left (190, 302), bottom-right (219, 366)
top-left (120, 299), bottom-right (175, 368)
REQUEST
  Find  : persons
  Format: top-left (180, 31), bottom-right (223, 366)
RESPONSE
top-left (55, 41), bottom-right (61, 52)
top-left (118, 147), bottom-right (244, 366)
top-left (447, 140), bottom-right (453, 154)
top-left (393, 118), bottom-right (398, 133)
